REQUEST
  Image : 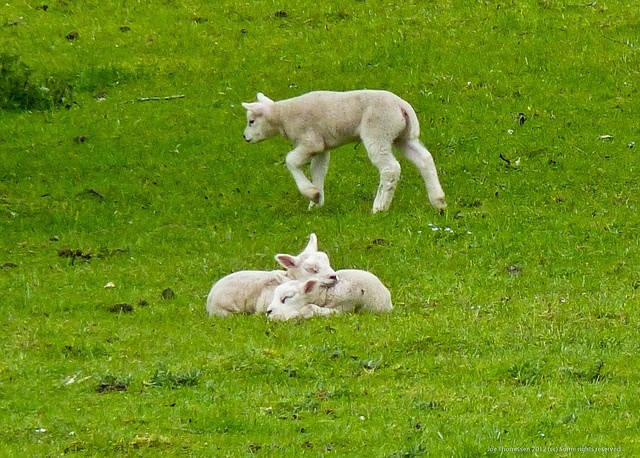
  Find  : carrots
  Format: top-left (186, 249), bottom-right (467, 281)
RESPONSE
top-left (206, 231), bottom-right (339, 318)
top-left (241, 88), bottom-right (447, 217)
top-left (265, 267), bottom-right (395, 321)
